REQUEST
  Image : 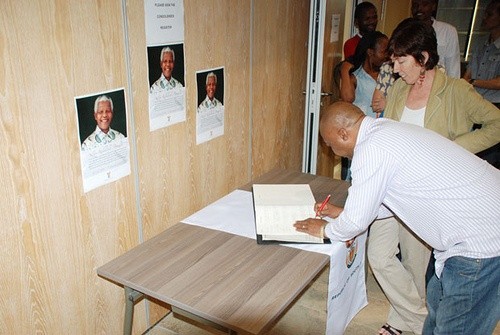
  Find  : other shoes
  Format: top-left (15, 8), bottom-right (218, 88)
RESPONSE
top-left (378, 323), bottom-right (402, 335)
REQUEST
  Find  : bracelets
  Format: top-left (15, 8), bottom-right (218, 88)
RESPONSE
top-left (321, 222), bottom-right (334, 240)
top-left (468, 79), bottom-right (476, 83)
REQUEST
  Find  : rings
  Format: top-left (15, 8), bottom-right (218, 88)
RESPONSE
top-left (302, 225), bottom-right (304, 229)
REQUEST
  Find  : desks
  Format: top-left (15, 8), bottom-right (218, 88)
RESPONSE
top-left (97, 169), bottom-right (356, 335)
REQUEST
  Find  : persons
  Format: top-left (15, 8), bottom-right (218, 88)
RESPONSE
top-left (366, 18), bottom-right (500, 335)
top-left (370, 38), bottom-right (402, 118)
top-left (198, 72), bottom-right (222, 110)
top-left (150, 47), bottom-right (184, 94)
top-left (411, 0), bottom-right (461, 80)
top-left (461, 0), bottom-right (500, 172)
top-left (333, 31), bottom-right (390, 120)
top-left (294, 102), bottom-right (500, 335)
top-left (345, 2), bottom-right (379, 61)
top-left (82, 95), bottom-right (126, 149)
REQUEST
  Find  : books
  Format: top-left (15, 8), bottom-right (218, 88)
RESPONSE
top-left (252, 183), bottom-right (331, 246)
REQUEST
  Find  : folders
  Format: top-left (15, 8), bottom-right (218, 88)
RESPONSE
top-left (252, 184), bottom-right (332, 245)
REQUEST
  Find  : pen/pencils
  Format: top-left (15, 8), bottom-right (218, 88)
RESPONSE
top-left (315, 195), bottom-right (331, 217)
